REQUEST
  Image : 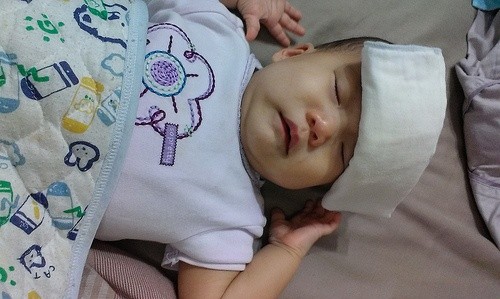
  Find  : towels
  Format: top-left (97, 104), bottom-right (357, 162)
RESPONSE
top-left (322, 41), bottom-right (448, 219)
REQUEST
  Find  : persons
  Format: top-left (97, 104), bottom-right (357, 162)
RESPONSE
top-left (1, 0), bottom-right (429, 297)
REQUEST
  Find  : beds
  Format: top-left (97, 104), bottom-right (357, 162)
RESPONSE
top-left (77, 0), bottom-right (500, 299)
